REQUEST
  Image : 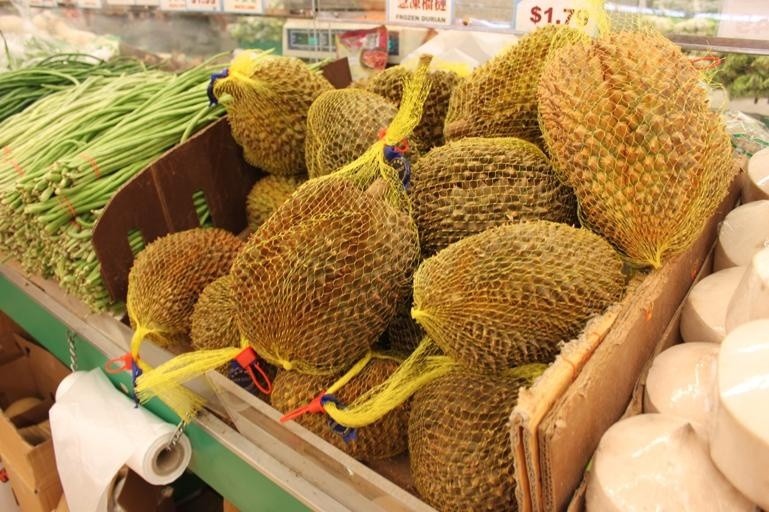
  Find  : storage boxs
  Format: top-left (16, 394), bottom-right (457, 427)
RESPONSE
top-left (109, 465), bottom-right (239, 512)
top-left (0, 334), bottom-right (72, 492)
top-left (4, 459), bottom-right (62, 512)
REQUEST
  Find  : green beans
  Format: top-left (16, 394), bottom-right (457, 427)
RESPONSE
top-left (0, 47), bottom-right (335, 316)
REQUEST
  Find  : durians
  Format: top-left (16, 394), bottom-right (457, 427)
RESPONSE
top-left (128, 28), bottom-right (731, 512)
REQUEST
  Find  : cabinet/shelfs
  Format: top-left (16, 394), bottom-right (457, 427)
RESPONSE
top-left (0, 17), bottom-right (436, 512)
